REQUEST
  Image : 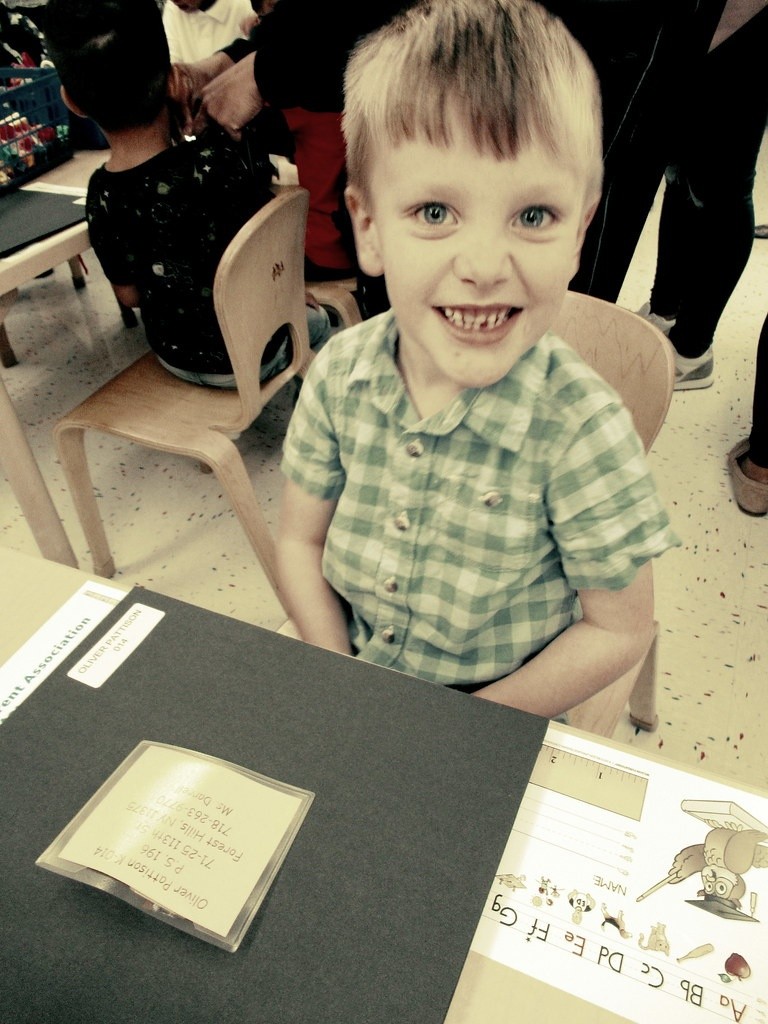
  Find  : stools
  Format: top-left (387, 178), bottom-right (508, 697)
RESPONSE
top-left (303, 277), bottom-right (363, 328)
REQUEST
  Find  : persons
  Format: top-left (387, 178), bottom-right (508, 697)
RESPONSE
top-left (40, 0), bottom-right (329, 409)
top-left (275, 1), bottom-right (683, 733)
top-left (727, 314), bottom-right (768, 516)
top-left (158, 0), bottom-right (768, 393)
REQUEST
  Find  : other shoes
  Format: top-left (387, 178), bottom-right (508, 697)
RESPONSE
top-left (728, 437), bottom-right (768, 517)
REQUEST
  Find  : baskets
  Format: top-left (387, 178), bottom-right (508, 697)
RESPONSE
top-left (0, 67), bottom-right (73, 196)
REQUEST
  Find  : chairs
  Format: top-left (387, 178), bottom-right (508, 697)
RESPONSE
top-left (549, 290), bottom-right (676, 740)
top-left (51, 187), bottom-right (318, 639)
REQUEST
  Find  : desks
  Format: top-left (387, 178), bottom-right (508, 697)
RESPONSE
top-left (1, 149), bottom-right (249, 570)
top-left (0, 543), bottom-right (768, 1024)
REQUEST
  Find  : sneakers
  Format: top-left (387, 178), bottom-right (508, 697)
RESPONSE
top-left (674, 342), bottom-right (714, 392)
top-left (634, 300), bottom-right (676, 335)
